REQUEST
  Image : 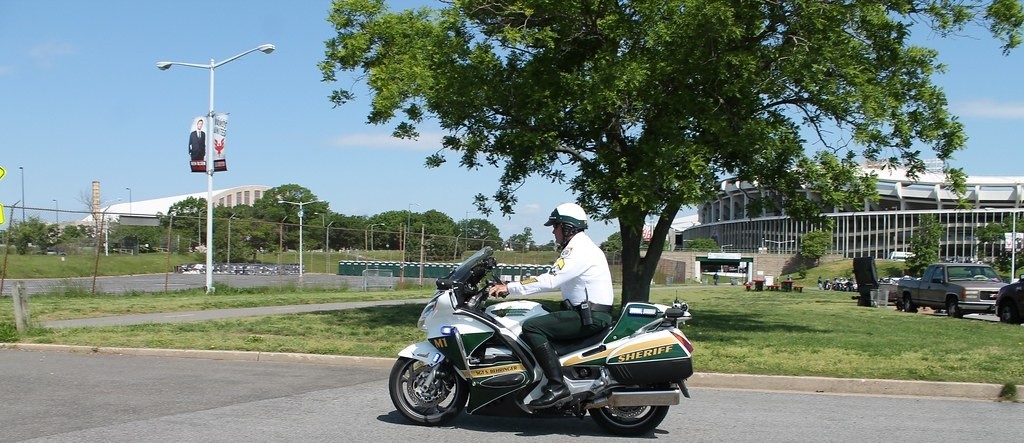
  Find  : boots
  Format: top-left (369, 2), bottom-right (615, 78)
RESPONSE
top-left (529, 342), bottom-right (574, 409)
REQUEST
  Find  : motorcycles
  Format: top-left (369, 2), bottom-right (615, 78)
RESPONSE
top-left (387, 247), bottom-right (695, 438)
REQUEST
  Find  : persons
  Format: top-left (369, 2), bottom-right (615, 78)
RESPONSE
top-left (189, 120), bottom-right (206, 161)
top-left (713, 273), bottom-right (718, 285)
top-left (788, 275), bottom-right (792, 280)
top-left (488, 203), bottom-right (614, 408)
top-left (818, 277), bottom-right (822, 290)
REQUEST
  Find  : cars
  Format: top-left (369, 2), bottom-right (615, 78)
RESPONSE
top-left (996, 274), bottom-right (1024, 324)
top-left (45, 249), bottom-right (67, 256)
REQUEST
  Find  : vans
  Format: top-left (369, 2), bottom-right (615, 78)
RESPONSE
top-left (888, 252), bottom-right (916, 263)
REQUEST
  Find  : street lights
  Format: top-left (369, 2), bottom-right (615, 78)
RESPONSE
top-left (720, 244), bottom-right (733, 253)
top-left (371, 223), bottom-right (386, 252)
top-left (154, 43), bottom-right (276, 297)
top-left (53, 199), bottom-right (59, 232)
top-left (314, 212), bottom-right (324, 232)
top-left (277, 200), bottom-right (324, 279)
top-left (408, 202), bottom-right (419, 245)
top-left (765, 239), bottom-right (795, 254)
top-left (19, 166), bottom-right (26, 221)
top-left (126, 187), bottom-right (132, 213)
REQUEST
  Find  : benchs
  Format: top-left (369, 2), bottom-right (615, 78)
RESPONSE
top-left (767, 285), bottom-right (780, 290)
top-left (794, 286), bottom-right (803, 293)
top-left (745, 284), bottom-right (751, 291)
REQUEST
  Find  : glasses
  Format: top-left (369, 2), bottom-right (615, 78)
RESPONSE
top-left (553, 224), bottom-right (559, 228)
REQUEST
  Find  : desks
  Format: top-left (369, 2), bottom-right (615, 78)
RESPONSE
top-left (781, 281), bottom-right (794, 292)
top-left (752, 280), bottom-right (765, 291)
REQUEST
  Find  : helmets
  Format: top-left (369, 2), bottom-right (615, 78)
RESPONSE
top-left (544, 202), bottom-right (588, 229)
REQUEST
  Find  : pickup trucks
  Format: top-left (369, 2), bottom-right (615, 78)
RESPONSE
top-left (895, 262), bottom-right (1010, 318)
